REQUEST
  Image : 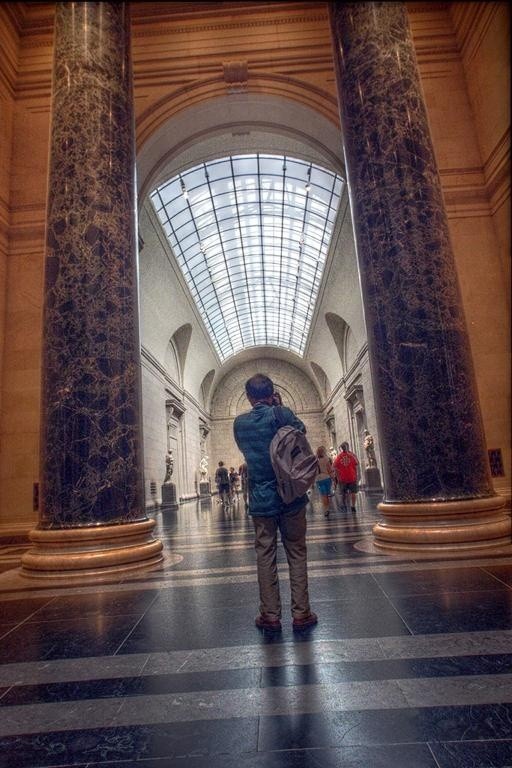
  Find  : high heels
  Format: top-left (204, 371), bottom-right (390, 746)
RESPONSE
top-left (324, 510), bottom-right (331, 517)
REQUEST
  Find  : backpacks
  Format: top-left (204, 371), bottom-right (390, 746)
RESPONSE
top-left (269, 406), bottom-right (321, 504)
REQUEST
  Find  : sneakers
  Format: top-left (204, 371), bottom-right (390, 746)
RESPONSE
top-left (351, 506), bottom-right (357, 512)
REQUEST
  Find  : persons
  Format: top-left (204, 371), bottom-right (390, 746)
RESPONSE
top-left (233, 373), bottom-right (317, 631)
top-left (363, 429), bottom-right (377, 469)
top-left (164, 449), bottom-right (174, 483)
top-left (199, 455), bottom-right (209, 482)
top-left (215, 442), bottom-right (361, 516)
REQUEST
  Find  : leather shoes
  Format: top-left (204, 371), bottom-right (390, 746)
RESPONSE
top-left (255, 616), bottom-right (282, 633)
top-left (292, 612), bottom-right (318, 630)
